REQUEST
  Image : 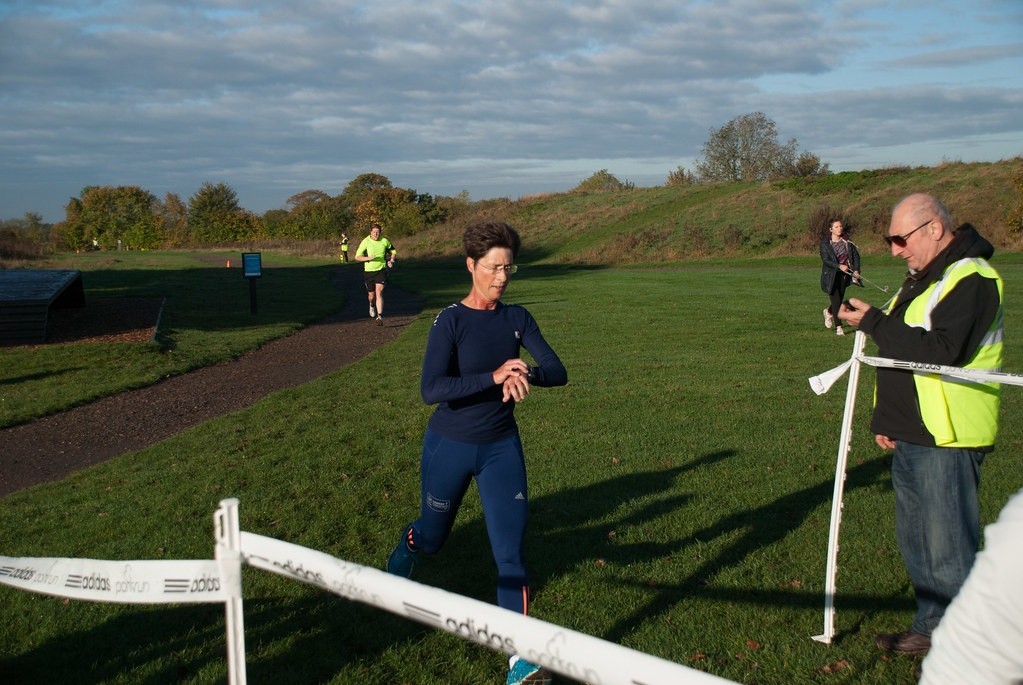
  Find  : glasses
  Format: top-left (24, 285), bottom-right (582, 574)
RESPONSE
top-left (475, 259), bottom-right (518, 274)
top-left (883, 219), bottom-right (934, 246)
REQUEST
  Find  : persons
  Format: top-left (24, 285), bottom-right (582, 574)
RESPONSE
top-left (835, 191), bottom-right (1001, 654)
top-left (356, 224), bottom-right (397, 325)
top-left (819, 218), bottom-right (863, 337)
top-left (383, 221), bottom-right (575, 685)
top-left (93, 236), bottom-right (99, 250)
top-left (339, 232), bottom-right (349, 264)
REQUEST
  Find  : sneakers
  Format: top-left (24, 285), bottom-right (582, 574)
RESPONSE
top-left (504, 657), bottom-right (552, 685)
top-left (823, 308), bottom-right (834, 328)
top-left (836, 325), bottom-right (844, 335)
top-left (375, 315), bottom-right (383, 325)
top-left (369, 303), bottom-right (376, 317)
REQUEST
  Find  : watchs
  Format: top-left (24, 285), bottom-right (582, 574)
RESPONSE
top-left (518, 360), bottom-right (533, 382)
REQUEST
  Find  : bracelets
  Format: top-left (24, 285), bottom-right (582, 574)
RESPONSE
top-left (391, 258), bottom-right (395, 263)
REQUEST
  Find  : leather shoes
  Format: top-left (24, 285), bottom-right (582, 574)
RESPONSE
top-left (876, 628), bottom-right (932, 653)
